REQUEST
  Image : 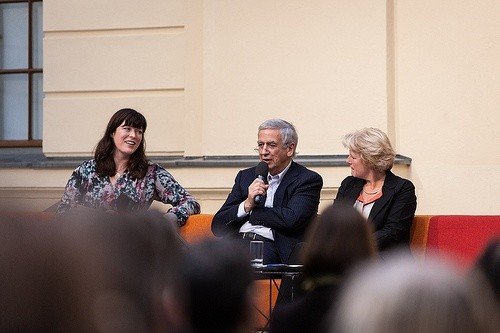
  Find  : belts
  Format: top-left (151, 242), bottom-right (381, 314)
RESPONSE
top-left (237, 231), bottom-right (275, 244)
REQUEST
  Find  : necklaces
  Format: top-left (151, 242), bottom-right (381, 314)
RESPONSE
top-left (363, 174), bottom-right (385, 195)
top-left (114, 164), bottom-right (127, 181)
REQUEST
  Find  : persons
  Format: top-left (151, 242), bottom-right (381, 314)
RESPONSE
top-left (1, 196), bottom-right (500, 333)
top-left (55, 108), bottom-right (201, 234)
top-left (331, 127), bottom-right (417, 259)
top-left (211, 120), bottom-right (324, 280)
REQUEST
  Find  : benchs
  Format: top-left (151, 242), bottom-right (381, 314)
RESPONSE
top-left (174, 211), bottom-right (500, 328)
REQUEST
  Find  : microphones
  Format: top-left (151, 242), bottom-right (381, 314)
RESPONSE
top-left (254, 162), bottom-right (268, 204)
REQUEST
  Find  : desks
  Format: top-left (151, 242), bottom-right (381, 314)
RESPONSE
top-left (235, 266), bottom-right (308, 333)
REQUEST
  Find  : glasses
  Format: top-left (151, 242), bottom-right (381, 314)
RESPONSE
top-left (254, 142), bottom-right (290, 153)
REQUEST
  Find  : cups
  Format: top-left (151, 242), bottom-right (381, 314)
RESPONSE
top-left (250, 241), bottom-right (264, 269)
